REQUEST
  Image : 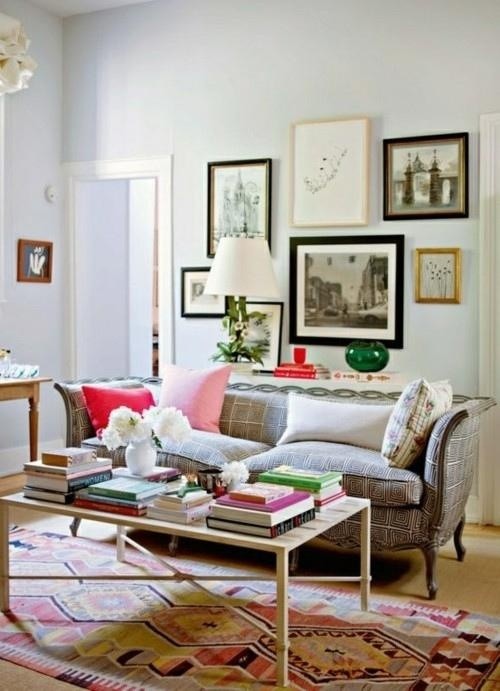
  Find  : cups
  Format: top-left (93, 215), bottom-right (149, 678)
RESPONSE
top-left (293, 347), bottom-right (306, 364)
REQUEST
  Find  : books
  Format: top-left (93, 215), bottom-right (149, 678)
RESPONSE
top-left (274, 363), bottom-right (331, 380)
top-left (24, 448), bottom-right (216, 524)
top-left (206, 465), bottom-right (347, 538)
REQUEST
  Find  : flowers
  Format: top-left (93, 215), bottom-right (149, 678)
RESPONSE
top-left (209, 295), bottom-right (272, 361)
top-left (102, 407), bottom-right (188, 445)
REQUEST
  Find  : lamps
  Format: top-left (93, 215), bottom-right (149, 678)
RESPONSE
top-left (203, 236), bottom-right (282, 299)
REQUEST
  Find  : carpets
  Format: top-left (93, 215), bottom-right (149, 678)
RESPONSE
top-left (0, 524), bottom-right (499, 691)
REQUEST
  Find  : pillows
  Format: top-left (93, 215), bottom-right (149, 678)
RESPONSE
top-left (98, 429), bottom-right (273, 474)
top-left (79, 384), bottom-right (156, 441)
top-left (157, 363), bottom-right (235, 434)
top-left (276, 393), bottom-right (400, 452)
top-left (237, 442), bottom-right (422, 510)
top-left (380, 377), bottom-right (454, 469)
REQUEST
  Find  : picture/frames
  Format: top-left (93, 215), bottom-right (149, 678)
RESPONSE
top-left (180, 266), bottom-right (227, 320)
top-left (15, 239), bottom-right (53, 285)
top-left (380, 132), bottom-right (472, 219)
top-left (291, 115), bottom-right (371, 230)
top-left (413, 246), bottom-right (461, 307)
top-left (207, 157), bottom-right (273, 258)
top-left (289, 234), bottom-right (404, 349)
top-left (228, 300), bottom-right (285, 373)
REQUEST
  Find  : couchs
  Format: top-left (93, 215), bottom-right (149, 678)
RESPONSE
top-left (53, 375), bottom-right (497, 601)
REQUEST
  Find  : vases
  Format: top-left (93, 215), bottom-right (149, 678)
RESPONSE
top-left (127, 441), bottom-right (154, 476)
top-left (236, 361), bottom-right (256, 374)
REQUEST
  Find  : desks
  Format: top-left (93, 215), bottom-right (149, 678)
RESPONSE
top-left (0, 378), bottom-right (54, 461)
top-left (0, 466), bottom-right (373, 687)
